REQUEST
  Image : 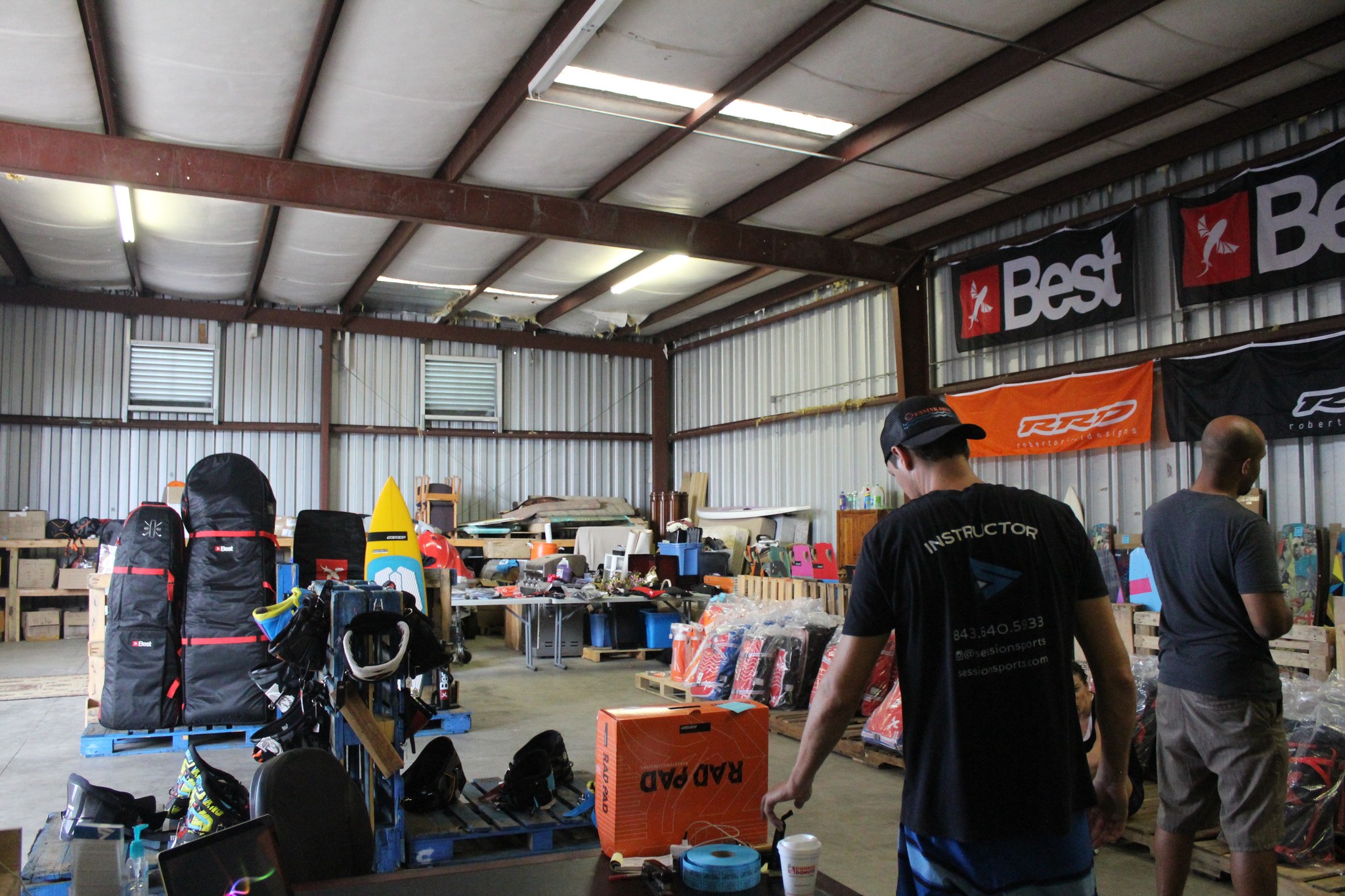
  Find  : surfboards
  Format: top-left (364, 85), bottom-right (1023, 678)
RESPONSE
top-left (1061, 486), bottom-right (1329, 624)
top-left (696, 505), bottom-right (812, 520)
top-left (364, 477), bottom-right (427, 616)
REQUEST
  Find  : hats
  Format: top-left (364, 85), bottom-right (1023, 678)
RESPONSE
top-left (880, 395), bottom-right (986, 465)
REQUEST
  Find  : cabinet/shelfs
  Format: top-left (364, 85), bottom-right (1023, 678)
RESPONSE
top-left (0, 539), bottom-right (577, 652)
top-left (836, 508), bottom-right (895, 570)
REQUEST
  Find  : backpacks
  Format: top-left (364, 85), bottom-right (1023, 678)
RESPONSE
top-left (176, 453), bottom-right (278, 730)
top-left (686, 594), bottom-right (904, 756)
top-left (43, 517), bottom-right (122, 550)
top-left (1084, 665), bottom-right (1161, 783)
top-left (98, 502), bottom-right (185, 730)
top-left (1272, 714), bottom-right (1343, 864)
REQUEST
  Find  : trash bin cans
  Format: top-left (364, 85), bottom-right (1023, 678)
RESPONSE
top-left (587, 613), bottom-right (611, 647)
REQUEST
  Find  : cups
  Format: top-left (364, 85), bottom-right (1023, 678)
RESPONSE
top-left (776, 834), bottom-right (821, 896)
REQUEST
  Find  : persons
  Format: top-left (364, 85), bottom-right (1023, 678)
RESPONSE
top-left (1070, 661), bottom-right (1145, 815)
top-left (1142, 414), bottom-right (1293, 896)
top-left (762, 396), bottom-right (1135, 896)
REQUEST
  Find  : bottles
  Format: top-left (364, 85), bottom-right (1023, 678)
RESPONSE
top-left (556, 557), bottom-right (572, 582)
top-left (839, 484), bottom-right (884, 511)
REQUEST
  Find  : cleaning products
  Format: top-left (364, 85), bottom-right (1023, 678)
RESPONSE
top-left (123, 823), bottom-right (151, 896)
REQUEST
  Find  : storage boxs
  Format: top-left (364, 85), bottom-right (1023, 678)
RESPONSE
top-left (1236, 487), bottom-right (1267, 517)
top-left (1113, 534), bottom-right (1144, 549)
top-left (0, 482), bottom-right (704, 650)
top-left (596, 697), bottom-right (772, 857)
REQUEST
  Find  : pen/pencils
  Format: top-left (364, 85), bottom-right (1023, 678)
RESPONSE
top-left (608, 870), bottom-right (677, 880)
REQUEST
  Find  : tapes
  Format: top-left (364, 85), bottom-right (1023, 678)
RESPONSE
top-left (681, 843), bottom-right (762, 893)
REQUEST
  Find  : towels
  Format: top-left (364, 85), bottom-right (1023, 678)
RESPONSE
top-left (666, 517), bottom-right (692, 533)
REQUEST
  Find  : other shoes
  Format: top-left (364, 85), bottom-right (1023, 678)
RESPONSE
top-left (401, 736), bottom-right (467, 812)
top-left (500, 730), bottom-right (574, 814)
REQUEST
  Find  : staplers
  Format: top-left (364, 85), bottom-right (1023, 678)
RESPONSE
top-left (639, 858), bottom-right (674, 896)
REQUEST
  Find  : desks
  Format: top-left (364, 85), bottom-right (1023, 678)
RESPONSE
top-left (450, 597), bottom-right (551, 673)
top-left (551, 592), bottom-right (712, 671)
